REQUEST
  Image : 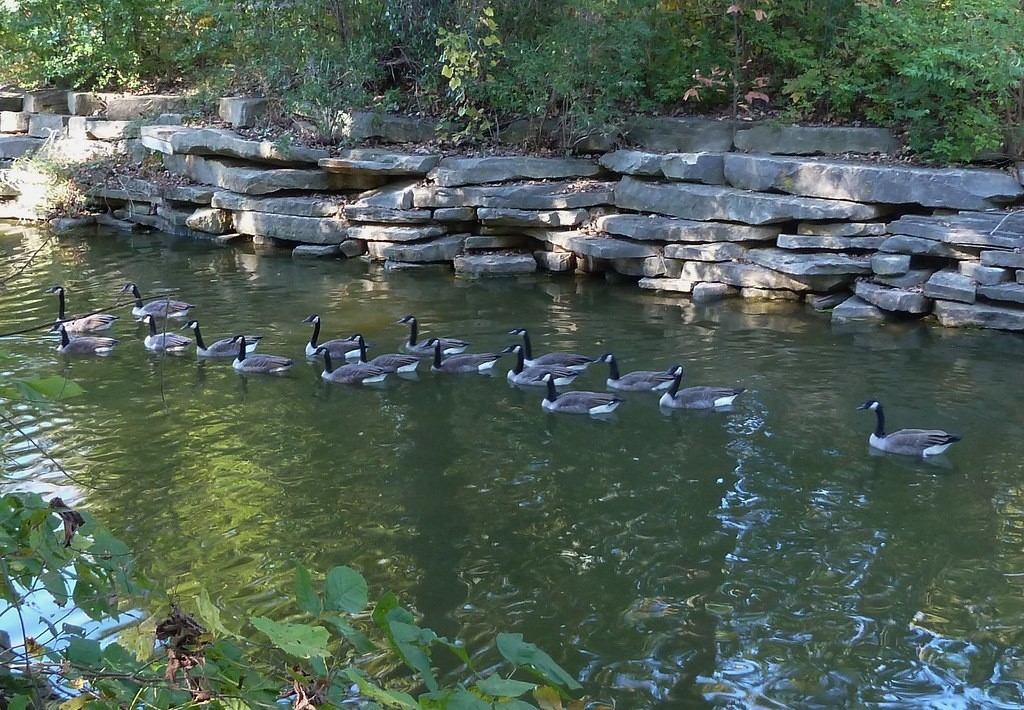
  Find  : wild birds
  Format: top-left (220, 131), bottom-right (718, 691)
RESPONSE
top-left (855, 398), bottom-right (963, 456)
top-left (44, 280), bottom-right (747, 415)
